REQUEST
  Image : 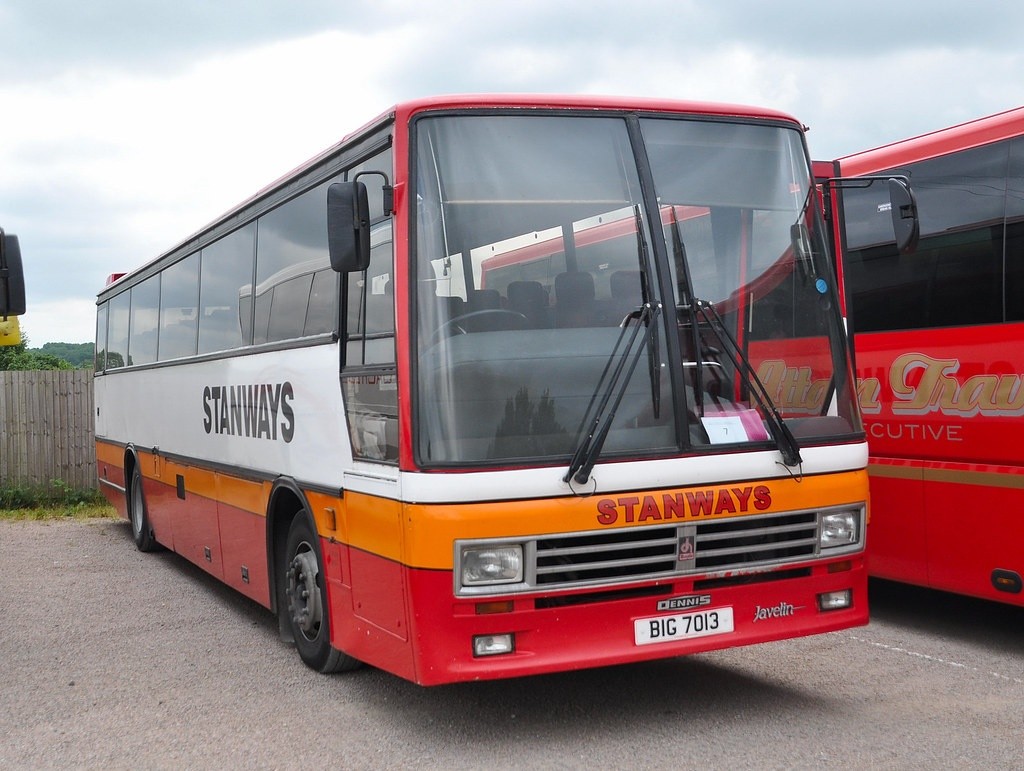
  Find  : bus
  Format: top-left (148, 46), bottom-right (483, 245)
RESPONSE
top-left (93, 94), bottom-right (921, 688)
top-left (481, 105), bottom-right (1024, 611)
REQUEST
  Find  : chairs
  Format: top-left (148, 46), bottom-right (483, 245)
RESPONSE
top-left (507, 280), bottom-right (549, 307)
top-left (470, 289), bottom-right (502, 310)
top-left (611, 271), bottom-right (645, 299)
top-left (555, 272), bottom-right (595, 303)
